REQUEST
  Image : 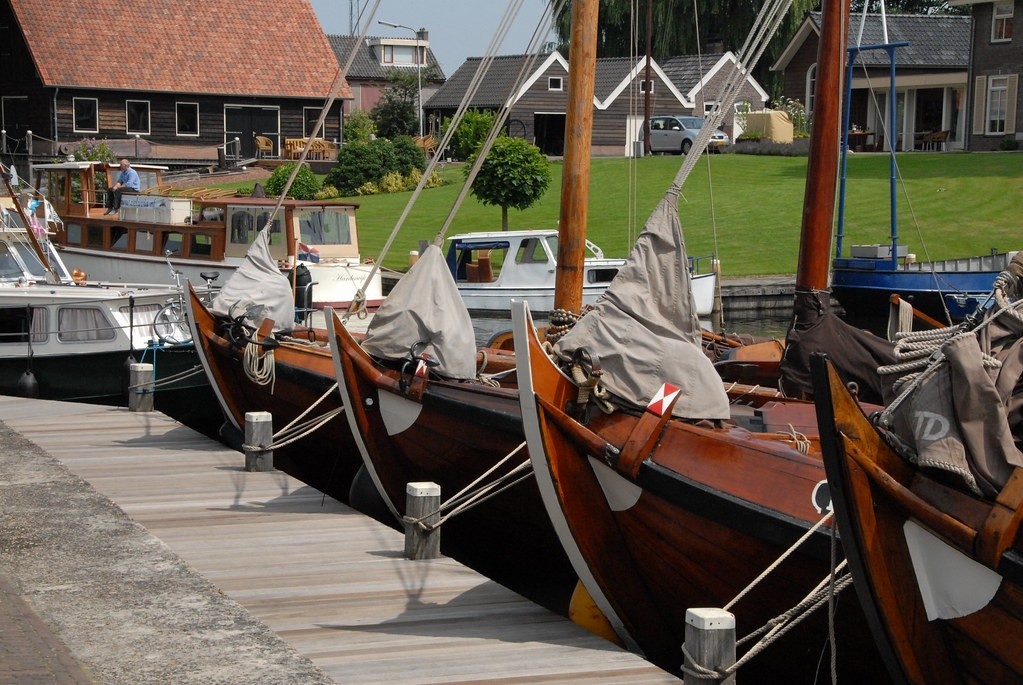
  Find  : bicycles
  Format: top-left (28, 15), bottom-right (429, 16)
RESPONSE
top-left (152, 248), bottom-right (222, 346)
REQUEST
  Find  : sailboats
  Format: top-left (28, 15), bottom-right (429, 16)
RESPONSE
top-left (183, 0), bottom-right (1023, 685)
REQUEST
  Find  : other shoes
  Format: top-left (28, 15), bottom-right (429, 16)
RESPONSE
top-left (104, 208), bottom-right (112, 215)
top-left (109, 207), bottom-right (118, 215)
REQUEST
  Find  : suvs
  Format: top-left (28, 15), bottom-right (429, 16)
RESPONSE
top-left (638, 116), bottom-right (730, 156)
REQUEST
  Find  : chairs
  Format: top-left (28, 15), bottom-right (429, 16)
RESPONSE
top-left (466, 256), bottom-right (491, 285)
top-left (141, 184), bottom-right (237, 218)
top-left (255, 136), bottom-right (273, 159)
top-left (413, 135), bottom-right (437, 156)
top-left (922, 130), bottom-right (950, 152)
top-left (285, 139), bottom-right (336, 160)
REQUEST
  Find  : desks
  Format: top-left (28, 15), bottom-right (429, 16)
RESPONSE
top-left (899, 132), bottom-right (930, 151)
top-left (848, 132), bottom-right (875, 152)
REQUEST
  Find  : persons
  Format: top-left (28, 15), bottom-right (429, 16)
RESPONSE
top-left (103, 158), bottom-right (140, 215)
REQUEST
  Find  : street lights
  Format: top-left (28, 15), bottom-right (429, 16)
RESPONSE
top-left (378, 21), bottom-right (424, 138)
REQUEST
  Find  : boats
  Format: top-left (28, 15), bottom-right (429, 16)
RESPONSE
top-left (381, 227), bottom-right (717, 319)
top-left (7, 153), bottom-right (361, 305)
top-left (0, 160), bottom-right (220, 410)
top-left (826, 0), bottom-right (1022, 338)
